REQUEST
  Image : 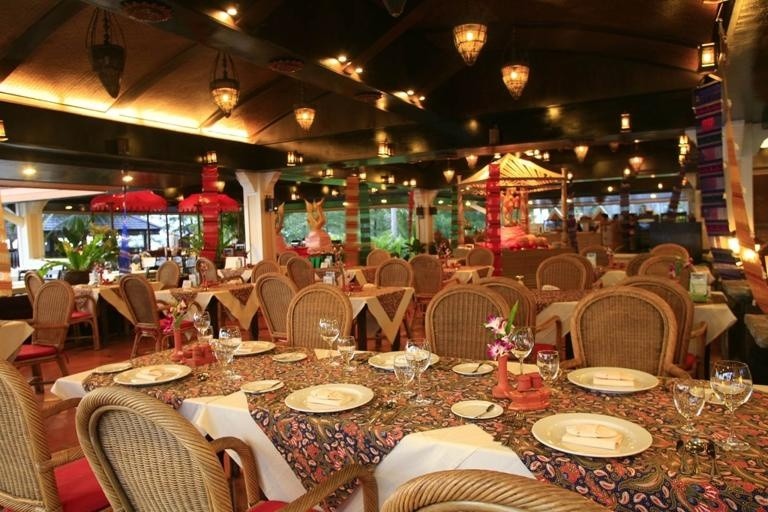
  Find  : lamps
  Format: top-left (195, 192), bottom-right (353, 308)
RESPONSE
top-left (499, 40), bottom-right (531, 101)
top-left (84, 1), bottom-right (127, 99)
top-left (697, 34), bottom-right (719, 74)
top-left (292, 78), bottom-right (317, 132)
top-left (450, 1), bottom-right (491, 69)
top-left (209, 46), bottom-right (241, 118)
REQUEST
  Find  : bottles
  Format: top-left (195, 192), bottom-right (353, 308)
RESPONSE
top-left (515, 276), bottom-right (526, 287)
top-left (453, 263), bottom-right (461, 271)
top-left (322, 272), bottom-right (336, 288)
top-left (199, 275), bottom-right (208, 291)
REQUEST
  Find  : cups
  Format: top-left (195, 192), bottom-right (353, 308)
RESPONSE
top-left (88, 273), bottom-right (100, 288)
top-left (681, 435), bottom-right (717, 479)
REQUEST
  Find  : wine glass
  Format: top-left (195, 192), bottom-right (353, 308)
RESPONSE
top-left (511, 327), bottom-right (560, 396)
top-left (191, 312), bottom-right (242, 377)
top-left (393, 338), bottom-right (433, 406)
top-left (319, 317), bottom-right (356, 371)
top-left (675, 361), bottom-right (754, 449)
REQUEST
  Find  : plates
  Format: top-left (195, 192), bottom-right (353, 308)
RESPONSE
top-left (451, 362), bottom-right (504, 420)
top-left (284, 383), bottom-right (374, 414)
top-left (367, 351), bottom-right (439, 370)
top-left (532, 367), bottom-right (660, 458)
top-left (235, 340), bottom-right (276, 356)
top-left (240, 352), bottom-right (308, 394)
top-left (92, 363), bottom-right (191, 385)
